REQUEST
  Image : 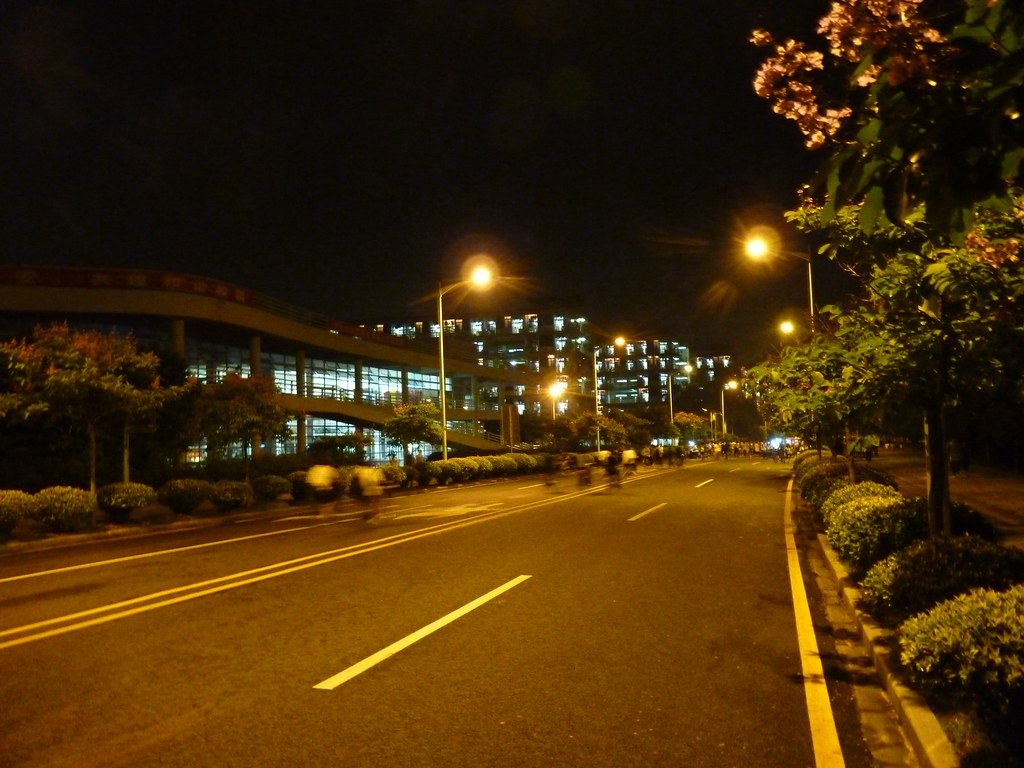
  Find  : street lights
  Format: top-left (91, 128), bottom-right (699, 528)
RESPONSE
top-left (438, 267), bottom-right (492, 461)
top-left (669, 365), bottom-right (693, 446)
top-left (722, 381), bottom-right (738, 444)
top-left (747, 239), bottom-right (815, 346)
top-left (593, 337), bottom-right (625, 452)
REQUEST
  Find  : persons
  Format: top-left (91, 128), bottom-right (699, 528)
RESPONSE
top-left (865, 433), bottom-right (880, 460)
top-left (544, 443), bottom-right (685, 490)
top-left (949, 438), bottom-right (964, 479)
top-left (771, 441), bottom-right (806, 464)
top-left (305, 457), bottom-right (383, 526)
top-left (417, 450), bottom-right (423, 463)
top-left (700, 440), bottom-right (764, 462)
